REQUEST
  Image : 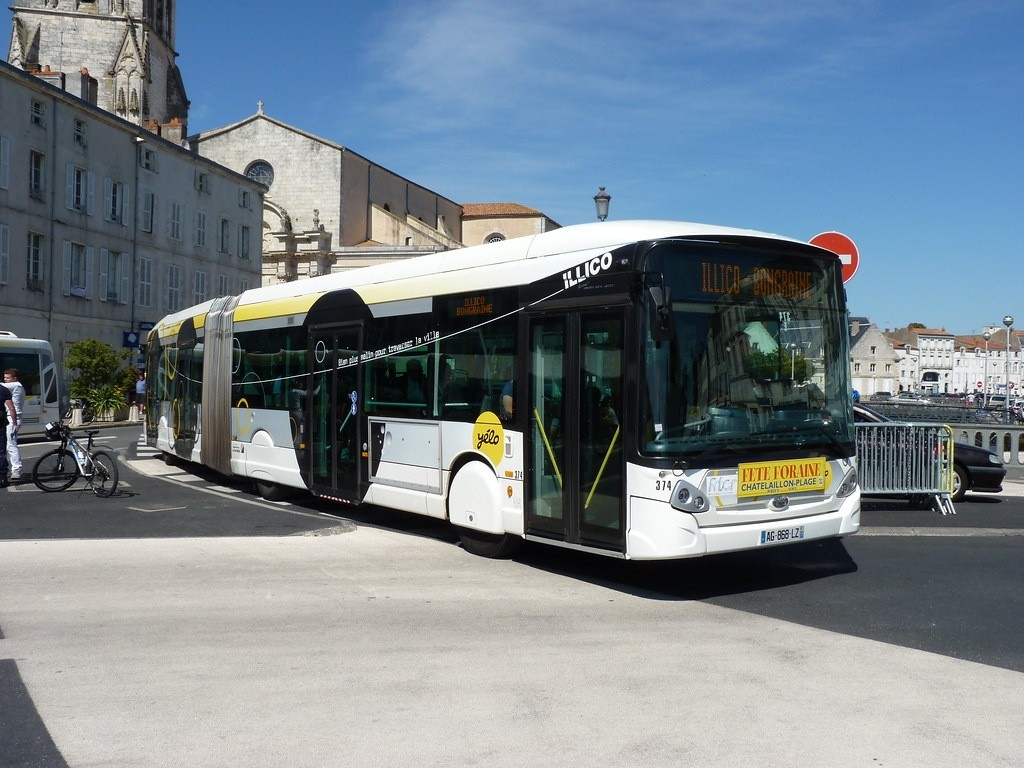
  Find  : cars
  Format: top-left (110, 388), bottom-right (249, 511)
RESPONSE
top-left (852, 390), bottom-right (860, 404)
top-left (887, 390), bottom-right (1024, 417)
top-left (853, 402), bottom-right (1008, 502)
top-left (869, 392), bottom-right (892, 401)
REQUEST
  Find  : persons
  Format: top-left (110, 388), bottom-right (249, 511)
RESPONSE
top-left (253, 354), bottom-right (486, 460)
top-left (0, 369), bottom-right (26, 489)
top-left (852, 387), bottom-right (860, 404)
top-left (698, 330), bottom-right (773, 409)
top-left (497, 348), bottom-right (657, 458)
top-left (136, 372), bottom-right (147, 415)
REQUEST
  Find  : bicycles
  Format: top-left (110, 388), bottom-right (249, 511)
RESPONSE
top-left (32, 420), bottom-right (118, 499)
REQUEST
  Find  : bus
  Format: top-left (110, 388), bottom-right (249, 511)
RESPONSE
top-left (0, 331), bottom-right (60, 439)
top-left (142, 219), bottom-right (858, 561)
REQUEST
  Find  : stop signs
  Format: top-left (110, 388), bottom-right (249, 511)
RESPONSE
top-left (1008, 381), bottom-right (1014, 389)
top-left (807, 231), bottom-right (859, 285)
top-left (977, 381), bottom-right (982, 387)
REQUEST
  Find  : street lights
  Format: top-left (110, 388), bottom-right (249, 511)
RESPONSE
top-left (593, 186), bottom-right (612, 222)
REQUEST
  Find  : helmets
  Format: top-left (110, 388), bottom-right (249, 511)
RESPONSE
top-left (45, 420), bottom-right (61, 441)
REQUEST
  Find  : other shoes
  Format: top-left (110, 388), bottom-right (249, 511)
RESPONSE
top-left (0, 479), bottom-right (8, 487)
top-left (139, 411), bottom-right (142, 415)
top-left (8, 478), bottom-right (21, 484)
